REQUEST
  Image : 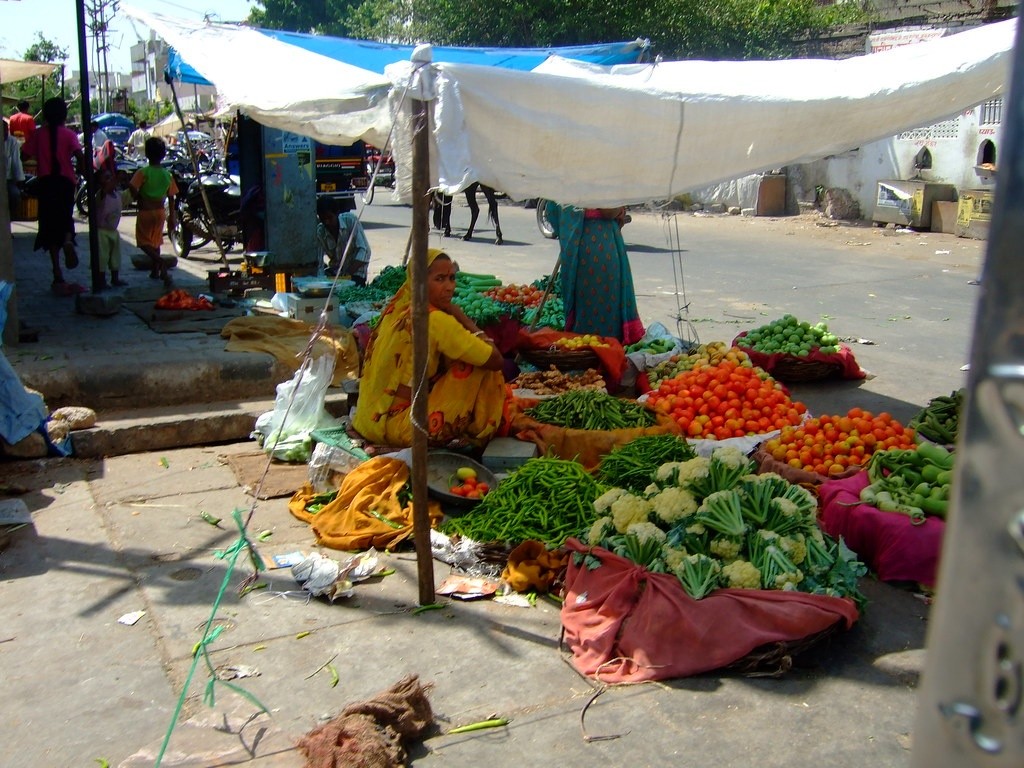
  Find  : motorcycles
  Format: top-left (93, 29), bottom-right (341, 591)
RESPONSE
top-left (536, 198), bottom-right (632, 238)
top-left (72, 110), bottom-right (398, 256)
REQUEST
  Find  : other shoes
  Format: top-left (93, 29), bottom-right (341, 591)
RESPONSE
top-left (160, 260), bottom-right (169, 280)
top-left (63, 239), bottom-right (79, 269)
top-left (103, 284), bottom-right (112, 289)
top-left (51, 280), bottom-right (66, 290)
top-left (149, 272), bottom-right (159, 279)
top-left (111, 280), bottom-right (129, 286)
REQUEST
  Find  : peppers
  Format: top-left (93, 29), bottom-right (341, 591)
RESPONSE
top-left (519, 387), bottom-right (698, 490)
top-left (191, 483), bottom-right (566, 733)
top-left (365, 314), bottom-right (382, 329)
top-left (160, 456), bottom-right (169, 468)
top-left (438, 445), bottom-right (616, 549)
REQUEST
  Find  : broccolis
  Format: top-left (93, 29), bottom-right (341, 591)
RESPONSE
top-left (584, 446), bottom-right (868, 627)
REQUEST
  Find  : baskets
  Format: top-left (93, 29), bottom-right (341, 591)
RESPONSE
top-left (557, 554), bottom-right (846, 679)
top-left (732, 332), bottom-right (838, 383)
top-left (520, 346), bottom-right (601, 374)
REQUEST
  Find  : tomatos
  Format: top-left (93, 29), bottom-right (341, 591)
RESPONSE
top-left (761, 407), bottom-right (917, 478)
top-left (550, 334), bottom-right (610, 348)
top-left (643, 360), bottom-right (808, 439)
top-left (482, 283), bottom-right (554, 309)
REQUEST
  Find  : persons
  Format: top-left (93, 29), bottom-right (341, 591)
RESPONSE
top-left (96, 159), bottom-right (138, 289)
top-left (0, 119), bottom-right (25, 222)
top-left (91, 120), bottom-right (109, 157)
top-left (20, 96), bottom-right (88, 288)
top-left (10, 99), bottom-right (36, 144)
top-left (125, 121), bottom-right (152, 158)
top-left (127, 136), bottom-right (180, 280)
top-left (315, 195), bottom-right (370, 286)
top-left (544, 198), bottom-right (646, 345)
top-left (349, 249), bottom-right (515, 450)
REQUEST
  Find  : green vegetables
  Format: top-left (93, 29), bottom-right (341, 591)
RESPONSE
top-left (337, 265), bottom-right (407, 303)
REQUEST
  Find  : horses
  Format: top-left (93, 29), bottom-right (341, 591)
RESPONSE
top-left (390, 161), bottom-right (503, 245)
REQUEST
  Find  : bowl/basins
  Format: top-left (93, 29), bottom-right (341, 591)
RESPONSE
top-left (428, 453), bottom-right (497, 505)
top-left (295, 281), bottom-right (341, 296)
